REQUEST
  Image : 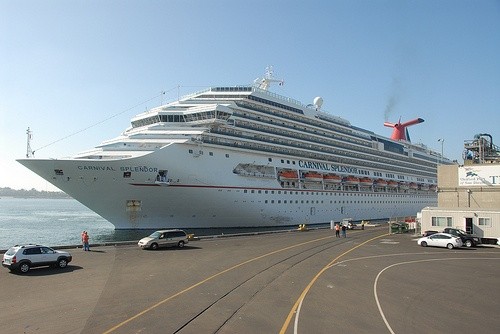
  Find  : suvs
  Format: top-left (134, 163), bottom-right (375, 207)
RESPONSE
top-left (422, 227), bottom-right (482, 247)
top-left (2, 244), bottom-right (72, 272)
top-left (138, 230), bottom-right (189, 250)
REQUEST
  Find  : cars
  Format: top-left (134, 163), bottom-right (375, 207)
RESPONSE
top-left (417, 233), bottom-right (463, 250)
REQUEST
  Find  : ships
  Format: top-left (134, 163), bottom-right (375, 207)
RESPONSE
top-left (16, 65), bottom-right (459, 230)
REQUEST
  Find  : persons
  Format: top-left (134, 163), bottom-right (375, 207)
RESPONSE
top-left (335, 223), bottom-right (340, 237)
top-left (82, 231), bottom-right (86, 244)
top-left (342, 225), bottom-right (346, 238)
top-left (83, 231), bottom-right (91, 251)
